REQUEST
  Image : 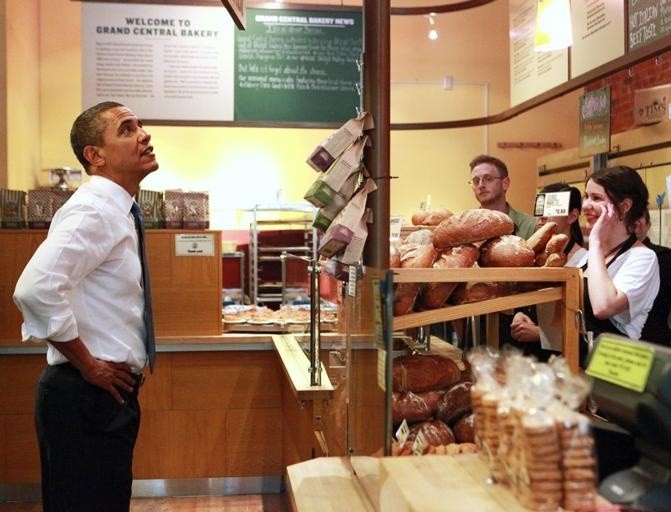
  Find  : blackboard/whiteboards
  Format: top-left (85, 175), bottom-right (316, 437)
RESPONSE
top-left (577, 85), bottom-right (613, 161)
top-left (625, 0), bottom-right (670, 53)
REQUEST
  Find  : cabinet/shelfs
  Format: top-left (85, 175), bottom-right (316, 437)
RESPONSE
top-left (247, 203), bottom-right (321, 306)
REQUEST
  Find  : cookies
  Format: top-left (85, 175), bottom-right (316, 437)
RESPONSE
top-left (468, 385), bottom-right (596, 511)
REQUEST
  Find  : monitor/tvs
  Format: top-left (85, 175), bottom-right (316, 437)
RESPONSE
top-left (585, 332), bottom-right (671, 500)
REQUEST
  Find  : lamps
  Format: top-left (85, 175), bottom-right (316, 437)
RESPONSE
top-left (535, 0), bottom-right (573, 52)
top-left (426, 13), bottom-right (439, 39)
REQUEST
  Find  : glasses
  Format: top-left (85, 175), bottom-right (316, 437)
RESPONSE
top-left (468, 176), bottom-right (500, 186)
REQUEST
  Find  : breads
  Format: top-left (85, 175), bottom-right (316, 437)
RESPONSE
top-left (390, 201), bottom-right (569, 315)
top-left (388, 354), bottom-right (508, 444)
top-left (223, 305), bottom-right (335, 322)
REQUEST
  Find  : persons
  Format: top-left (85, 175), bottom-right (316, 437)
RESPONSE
top-left (628, 205), bottom-right (671, 347)
top-left (574, 165), bottom-right (661, 371)
top-left (509, 180), bottom-right (589, 369)
top-left (457, 155), bottom-right (539, 351)
top-left (13, 101), bottom-right (159, 511)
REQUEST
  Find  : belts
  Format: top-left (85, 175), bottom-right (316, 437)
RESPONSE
top-left (126, 375), bottom-right (146, 391)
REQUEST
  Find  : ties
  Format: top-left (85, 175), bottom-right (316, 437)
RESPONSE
top-left (131, 204), bottom-right (157, 375)
